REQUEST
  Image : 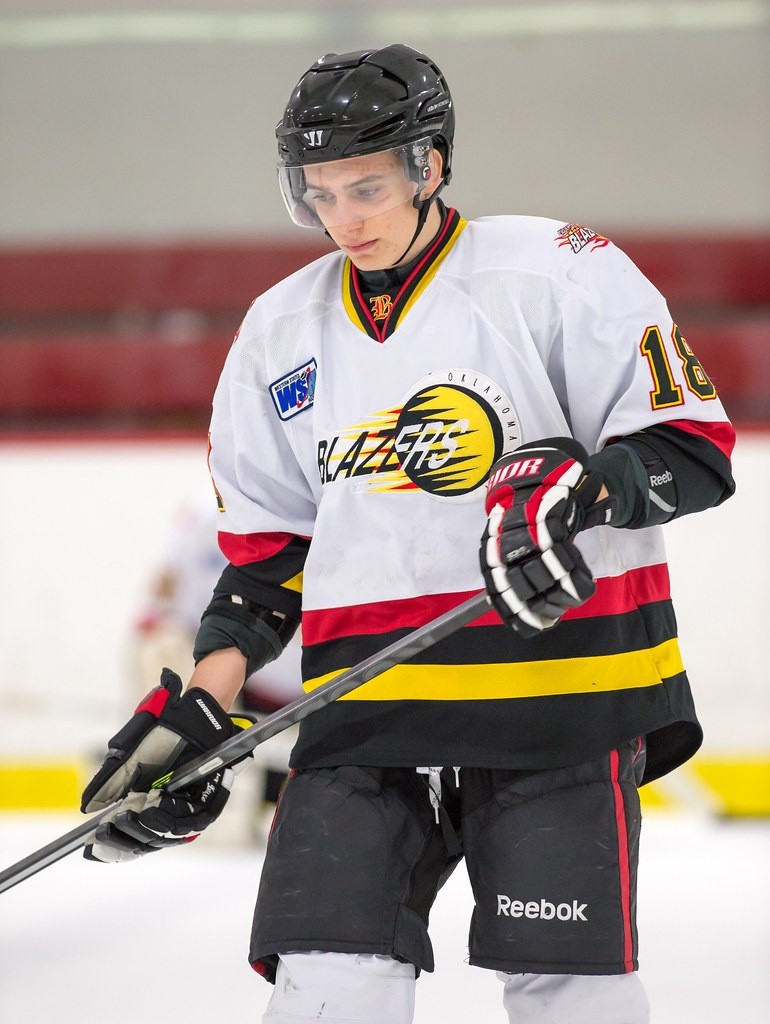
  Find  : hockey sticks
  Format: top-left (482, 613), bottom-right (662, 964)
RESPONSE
top-left (0, 587), bottom-right (495, 892)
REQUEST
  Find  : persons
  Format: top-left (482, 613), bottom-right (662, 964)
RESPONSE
top-left (78, 41), bottom-right (737, 1023)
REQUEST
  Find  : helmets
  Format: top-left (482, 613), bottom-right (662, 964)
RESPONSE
top-left (274, 43), bottom-right (456, 199)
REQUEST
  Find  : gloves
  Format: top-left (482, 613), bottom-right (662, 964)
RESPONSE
top-left (81, 668), bottom-right (258, 864)
top-left (480, 438), bottom-right (618, 639)
